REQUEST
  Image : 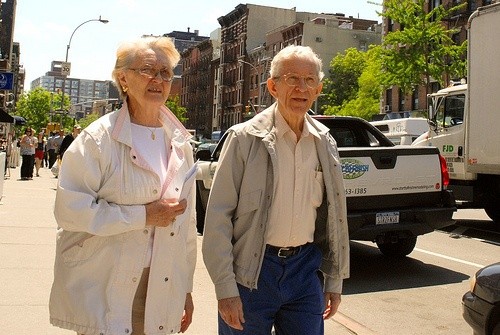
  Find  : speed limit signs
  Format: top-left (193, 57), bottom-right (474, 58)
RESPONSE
top-left (61, 62), bottom-right (71, 75)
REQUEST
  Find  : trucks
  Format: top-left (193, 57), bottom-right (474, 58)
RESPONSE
top-left (410, 1), bottom-right (500, 228)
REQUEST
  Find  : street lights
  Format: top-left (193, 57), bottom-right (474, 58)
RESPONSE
top-left (238, 60), bottom-right (261, 114)
top-left (57, 15), bottom-right (110, 162)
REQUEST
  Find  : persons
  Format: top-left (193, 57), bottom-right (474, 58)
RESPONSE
top-left (0, 121), bottom-right (81, 181)
top-left (49, 39), bottom-right (197, 335)
top-left (202, 45), bottom-right (351, 335)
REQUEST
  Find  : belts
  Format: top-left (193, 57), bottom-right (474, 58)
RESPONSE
top-left (265, 243), bottom-right (313, 258)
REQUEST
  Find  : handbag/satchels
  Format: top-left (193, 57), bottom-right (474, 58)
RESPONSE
top-left (51, 159), bottom-right (62, 176)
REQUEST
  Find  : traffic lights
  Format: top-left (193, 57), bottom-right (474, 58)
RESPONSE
top-left (115, 101), bottom-right (119, 109)
top-left (248, 106), bottom-right (251, 112)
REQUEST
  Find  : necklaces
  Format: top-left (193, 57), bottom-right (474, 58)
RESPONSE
top-left (137, 118), bottom-right (159, 140)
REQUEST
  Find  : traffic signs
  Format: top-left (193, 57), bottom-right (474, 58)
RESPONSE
top-left (55, 110), bottom-right (69, 114)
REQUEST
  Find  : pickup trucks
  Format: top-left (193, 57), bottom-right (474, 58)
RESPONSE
top-left (194, 115), bottom-right (459, 257)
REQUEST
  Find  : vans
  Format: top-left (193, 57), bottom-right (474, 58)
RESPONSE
top-left (367, 117), bottom-right (429, 146)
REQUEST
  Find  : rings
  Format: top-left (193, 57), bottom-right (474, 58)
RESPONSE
top-left (172, 219), bottom-right (177, 223)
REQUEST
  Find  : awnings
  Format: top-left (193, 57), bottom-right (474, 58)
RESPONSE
top-left (0, 108), bottom-right (26, 126)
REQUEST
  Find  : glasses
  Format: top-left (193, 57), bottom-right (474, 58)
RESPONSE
top-left (274, 73), bottom-right (320, 89)
top-left (127, 63), bottom-right (175, 82)
